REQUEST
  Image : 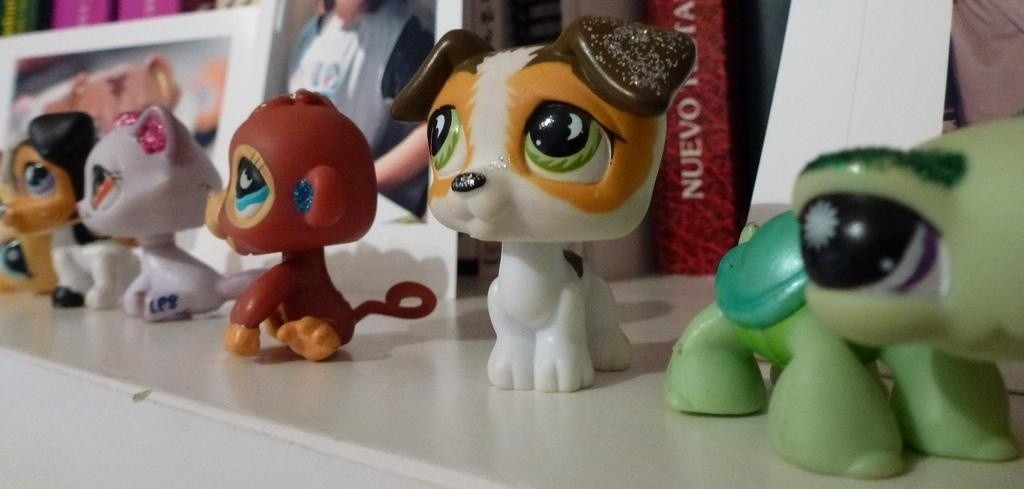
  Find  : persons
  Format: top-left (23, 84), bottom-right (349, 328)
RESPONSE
top-left (280, 1), bottom-right (435, 219)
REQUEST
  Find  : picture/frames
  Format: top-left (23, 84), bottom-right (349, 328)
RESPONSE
top-left (239, 1), bottom-right (462, 303)
top-left (1, 11), bottom-right (257, 278)
